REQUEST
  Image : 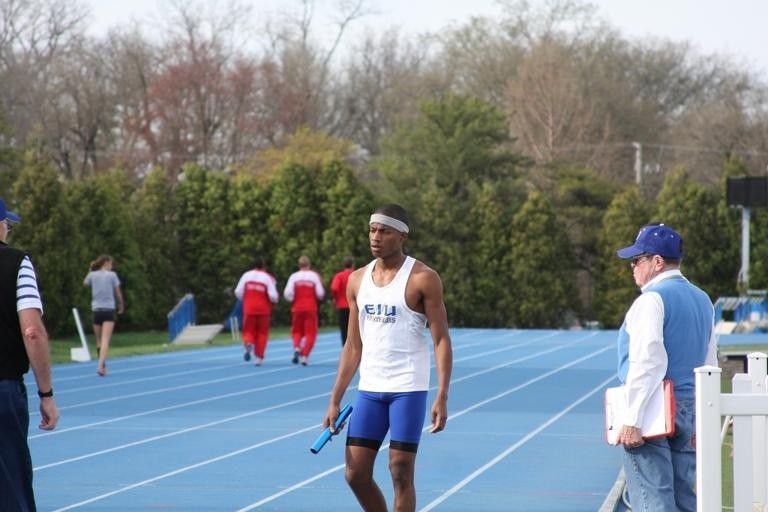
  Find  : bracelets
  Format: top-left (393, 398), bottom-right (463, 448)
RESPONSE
top-left (38, 388), bottom-right (53, 397)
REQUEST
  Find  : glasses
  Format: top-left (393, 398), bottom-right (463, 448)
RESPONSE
top-left (2, 222), bottom-right (13, 232)
top-left (632, 255), bottom-right (650, 264)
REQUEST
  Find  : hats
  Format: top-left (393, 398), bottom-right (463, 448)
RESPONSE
top-left (0, 199), bottom-right (18, 222)
top-left (617, 224), bottom-right (683, 258)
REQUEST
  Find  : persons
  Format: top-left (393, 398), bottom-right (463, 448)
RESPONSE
top-left (83, 255), bottom-right (124, 376)
top-left (233, 255), bottom-right (279, 366)
top-left (322, 204), bottom-right (452, 512)
top-left (283, 255), bottom-right (326, 366)
top-left (330, 256), bottom-right (355, 347)
top-left (616, 224), bottom-right (719, 511)
top-left (0, 208), bottom-right (58, 512)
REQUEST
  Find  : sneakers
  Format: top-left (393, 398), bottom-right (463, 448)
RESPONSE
top-left (292, 349), bottom-right (301, 363)
top-left (244, 344), bottom-right (253, 361)
